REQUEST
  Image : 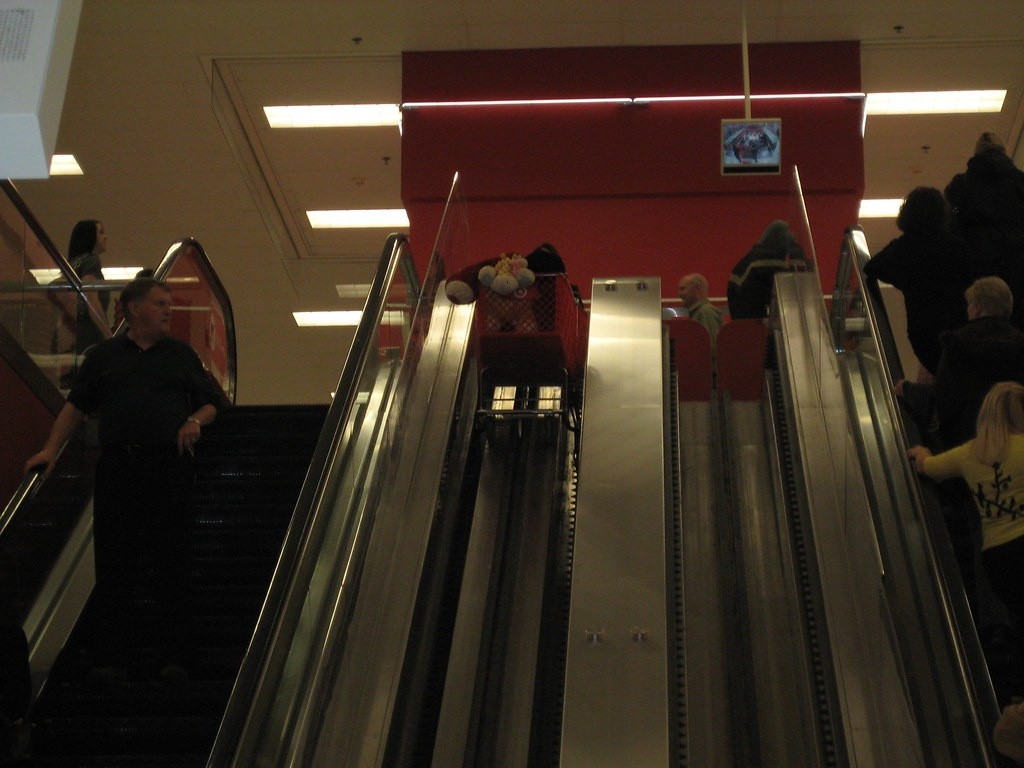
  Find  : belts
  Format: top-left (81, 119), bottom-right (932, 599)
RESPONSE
top-left (101, 445), bottom-right (179, 454)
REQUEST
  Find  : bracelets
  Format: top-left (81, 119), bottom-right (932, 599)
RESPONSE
top-left (185, 414), bottom-right (201, 424)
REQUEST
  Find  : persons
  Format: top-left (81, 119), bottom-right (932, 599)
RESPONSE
top-left (43, 220), bottom-right (155, 388)
top-left (24, 278), bottom-right (221, 681)
top-left (865, 132), bottom-right (1024, 767)
top-left (675, 273), bottom-right (723, 376)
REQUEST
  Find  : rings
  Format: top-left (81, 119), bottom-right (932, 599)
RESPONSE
top-left (191, 438), bottom-right (195, 441)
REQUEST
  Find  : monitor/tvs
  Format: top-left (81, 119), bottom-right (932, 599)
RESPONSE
top-left (720, 118), bottom-right (782, 176)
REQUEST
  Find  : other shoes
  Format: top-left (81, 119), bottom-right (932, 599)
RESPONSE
top-left (92, 667), bottom-right (129, 686)
top-left (159, 663), bottom-right (185, 683)
